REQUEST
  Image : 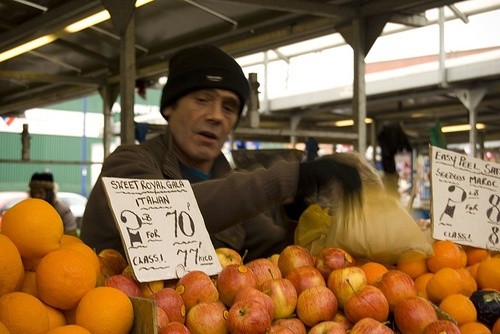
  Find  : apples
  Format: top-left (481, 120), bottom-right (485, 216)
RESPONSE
top-left (97, 245), bottom-right (126, 274)
top-left (153, 245), bottom-right (459, 334)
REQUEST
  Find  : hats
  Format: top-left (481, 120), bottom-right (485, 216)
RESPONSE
top-left (30, 173), bottom-right (54, 189)
top-left (160, 45), bottom-right (250, 122)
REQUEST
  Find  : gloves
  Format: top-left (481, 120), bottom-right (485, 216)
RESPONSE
top-left (298, 153), bottom-right (361, 199)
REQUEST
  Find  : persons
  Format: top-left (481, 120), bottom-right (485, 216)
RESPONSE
top-left (79, 44), bottom-right (362, 267)
top-left (29, 172), bottom-right (77, 235)
